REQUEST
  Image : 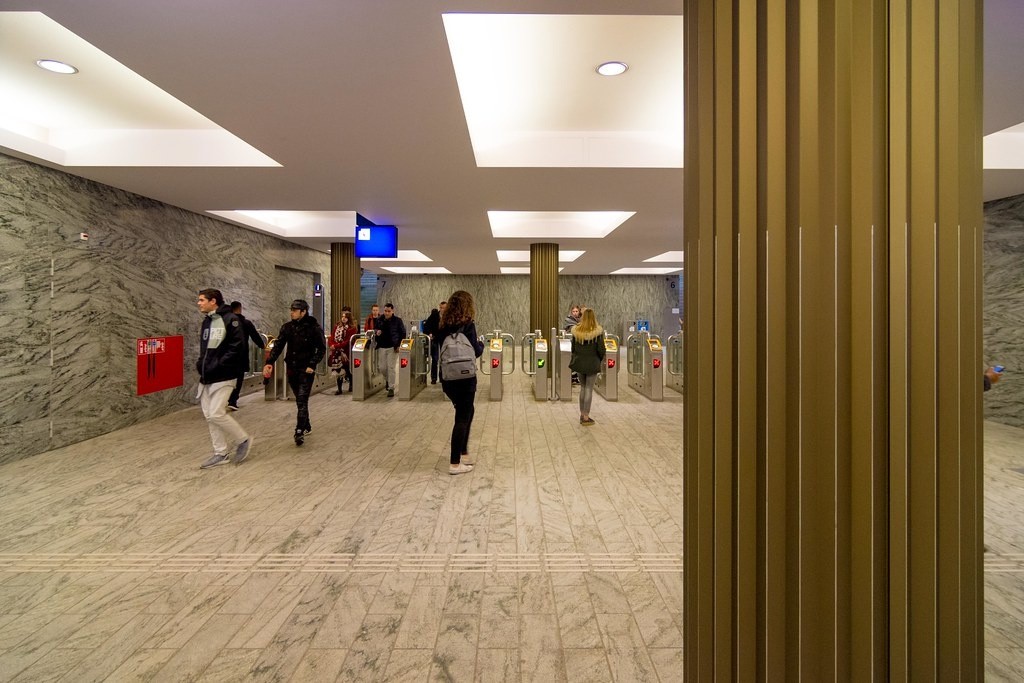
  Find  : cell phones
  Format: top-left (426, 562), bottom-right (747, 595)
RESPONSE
top-left (994, 365), bottom-right (1005, 372)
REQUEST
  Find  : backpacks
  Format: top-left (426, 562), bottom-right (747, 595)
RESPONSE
top-left (439, 322), bottom-right (478, 380)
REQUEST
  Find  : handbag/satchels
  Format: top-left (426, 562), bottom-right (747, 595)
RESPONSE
top-left (328, 349), bottom-right (348, 366)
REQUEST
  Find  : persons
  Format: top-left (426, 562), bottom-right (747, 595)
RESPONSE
top-left (563, 305), bottom-right (589, 385)
top-left (263, 299), bottom-right (326, 448)
top-left (364, 305), bottom-right (381, 332)
top-left (328, 306), bottom-right (360, 396)
top-left (423, 301), bottom-right (449, 385)
top-left (984, 366), bottom-right (1004, 554)
top-left (375, 303), bottom-right (406, 398)
top-left (569, 309), bottom-right (606, 427)
top-left (227, 301), bottom-right (266, 410)
top-left (438, 291), bottom-right (484, 476)
top-left (196, 288), bottom-right (253, 469)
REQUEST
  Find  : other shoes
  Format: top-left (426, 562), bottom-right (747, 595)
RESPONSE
top-left (461, 452), bottom-right (471, 462)
top-left (388, 389), bottom-right (395, 398)
top-left (572, 379), bottom-right (582, 386)
top-left (228, 403), bottom-right (240, 411)
top-left (199, 452), bottom-right (232, 469)
top-left (449, 463), bottom-right (473, 474)
top-left (235, 436), bottom-right (253, 464)
top-left (335, 390), bottom-right (341, 396)
top-left (294, 429), bottom-right (304, 445)
top-left (580, 416), bottom-right (595, 425)
top-left (304, 427), bottom-right (312, 435)
top-left (385, 381), bottom-right (388, 391)
